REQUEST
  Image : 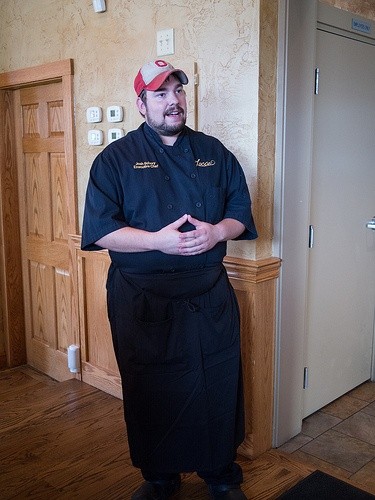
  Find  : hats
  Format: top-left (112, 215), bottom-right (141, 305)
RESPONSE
top-left (134, 59), bottom-right (189, 96)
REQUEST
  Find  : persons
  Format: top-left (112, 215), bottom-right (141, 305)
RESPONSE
top-left (80, 60), bottom-right (259, 500)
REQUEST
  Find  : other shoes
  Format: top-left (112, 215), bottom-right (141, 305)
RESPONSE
top-left (130, 475), bottom-right (181, 500)
top-left (207, 486), bottom-right (248, 500)
